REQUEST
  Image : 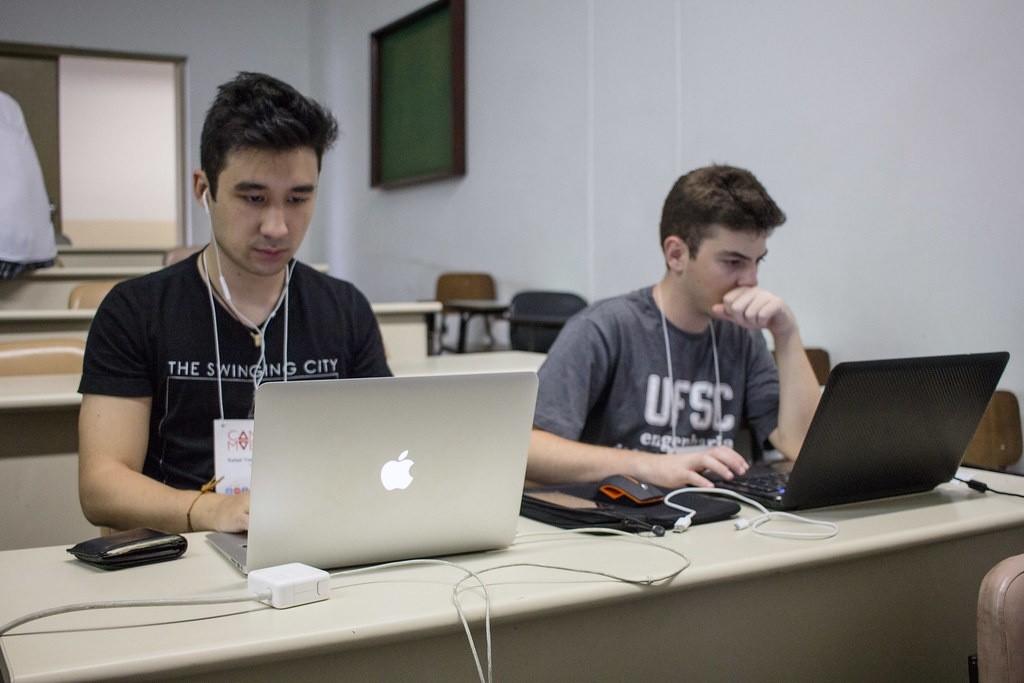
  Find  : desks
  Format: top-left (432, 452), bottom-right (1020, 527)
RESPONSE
top-left (0, 352), bottom-right (546, 550)
top-left (28, 264), bottom-right (330, 278)
top-left (0, 300), bottom-right (446, 332)
top-left (0, 462), bottom-right (1024, 683)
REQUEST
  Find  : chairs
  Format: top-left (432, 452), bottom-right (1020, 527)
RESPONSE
top-left (163, 245), bottom-right (203, 265)
top-left (962, 390), bottom-right (1024, 473)
top-left (432, 273), bottom-right (500, 351)
top-left (771, 347), bottom-right (829, 385)
top-left (508, 290), bottom-right (590, 353)
top-left (68, 280), bottom-right (120, 310)
top-left (0, 345), bottom-right (84, 376)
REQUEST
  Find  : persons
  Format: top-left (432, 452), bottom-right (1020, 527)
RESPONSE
top-left (525, 163), bottom-right (823, 487)
top-left (75, 69), bottom-right (396, 538)
top-left (0, 91), bottom-right (67, 281)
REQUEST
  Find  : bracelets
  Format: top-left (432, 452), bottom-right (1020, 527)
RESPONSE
top-left (185, 475), bottom-right (225, 533)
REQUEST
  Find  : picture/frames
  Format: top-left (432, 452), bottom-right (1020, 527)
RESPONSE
top-left (369, 0), bottom-right (468, 191)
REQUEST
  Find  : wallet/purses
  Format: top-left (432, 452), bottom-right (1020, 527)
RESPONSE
top-left (65, 526), bottom-right (188, 571)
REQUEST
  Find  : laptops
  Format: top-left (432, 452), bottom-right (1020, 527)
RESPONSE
top-left (688, 350), bottom-right (1009, 510)
top-left (207, 370), bottom-right (539, 576)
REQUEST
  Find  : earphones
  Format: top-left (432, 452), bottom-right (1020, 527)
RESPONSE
top-left (202, 188), bottom-right (212, 215)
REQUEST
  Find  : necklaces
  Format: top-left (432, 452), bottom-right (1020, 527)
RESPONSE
top-left (200, 241), bottom-right (287, 348)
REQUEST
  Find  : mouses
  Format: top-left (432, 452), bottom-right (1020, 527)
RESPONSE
top-left (592, 473), bottom-right (668, 507)
